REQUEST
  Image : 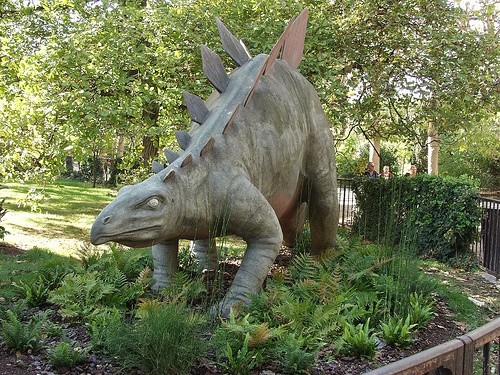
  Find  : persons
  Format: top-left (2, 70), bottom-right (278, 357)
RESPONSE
top-left (403, 165), bottom-right (417, 177)
top-left (380, 165), bottom-right (394, 180)
top-left (363, 161), bottom-right (379, 181)
